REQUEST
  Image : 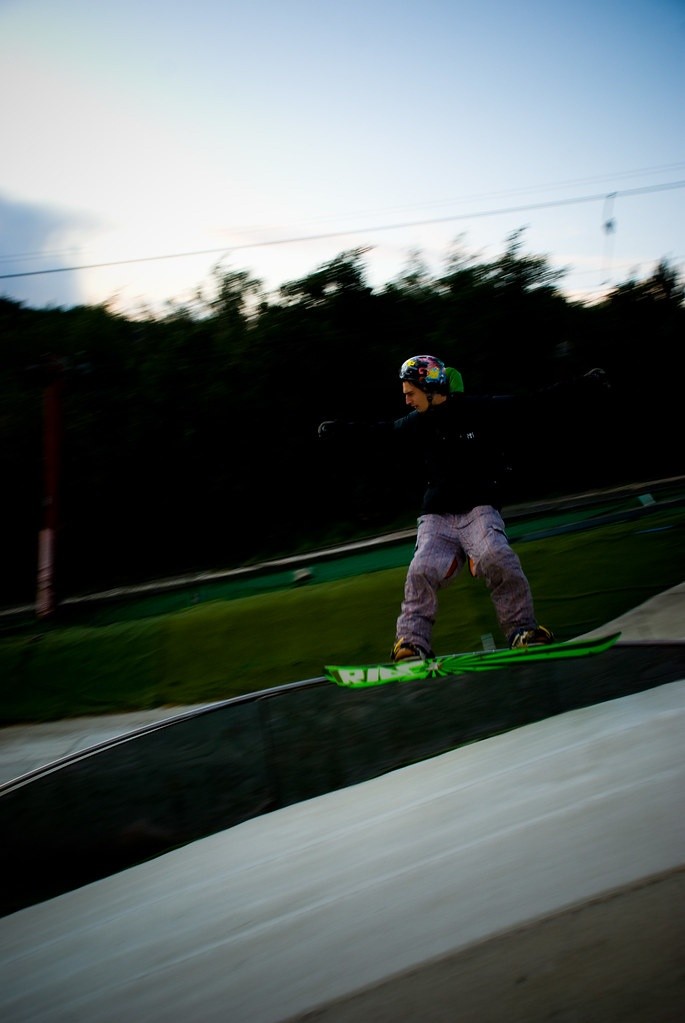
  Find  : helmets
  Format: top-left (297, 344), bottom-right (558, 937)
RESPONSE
top-left (399, 355), bottom-right (450, 392)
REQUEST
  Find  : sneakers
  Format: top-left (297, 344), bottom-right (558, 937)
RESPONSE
top-left (390, 637), bottom-right (433, 663)
top-left (512, 625), bottom-right (551, 648)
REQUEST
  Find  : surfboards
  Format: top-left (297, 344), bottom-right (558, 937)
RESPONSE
top-left (322, 630), bottom-right (623, 691)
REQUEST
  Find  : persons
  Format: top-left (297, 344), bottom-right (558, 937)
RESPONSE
top-left (316, 355), bottom-right (553, 662)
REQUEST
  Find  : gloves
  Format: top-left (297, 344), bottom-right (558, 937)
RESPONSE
top-left (317, 418), bottom-right (354, 441)
top-left (584, 367), bottom-right (605, 379)
